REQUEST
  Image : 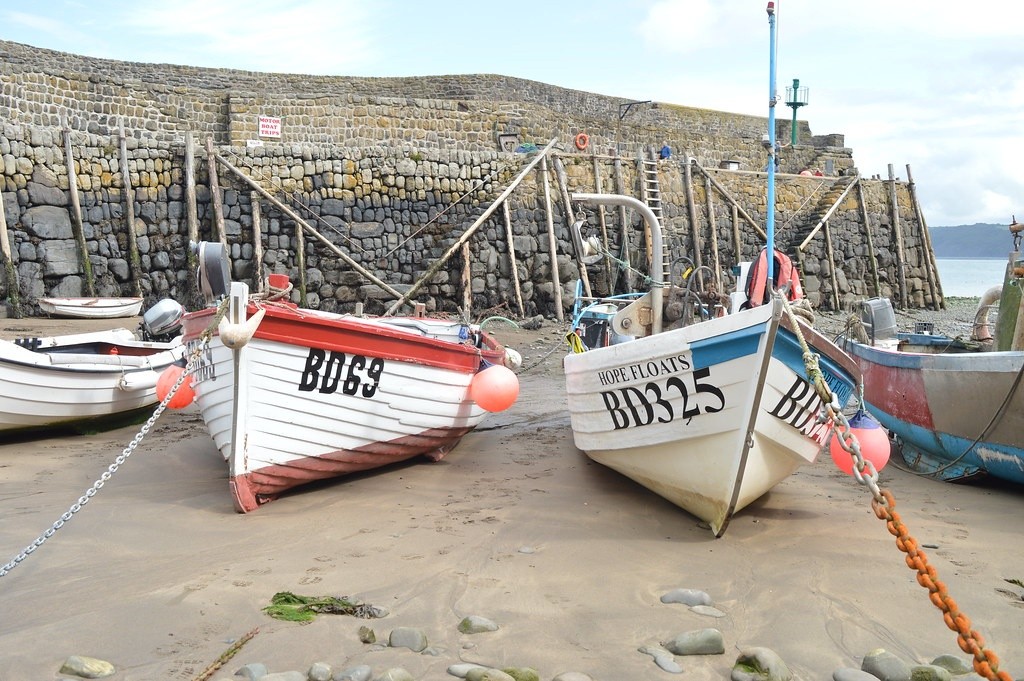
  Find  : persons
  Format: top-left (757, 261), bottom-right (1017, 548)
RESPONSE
top-left (837, 167), bottom-right (845, 176)
top-left (815, 168), bottom-right (823, 177)
top-left (775, 141), bottom-right (790, 156)
top-left (801, 167), bottom-right (813, 176)
top-left (660, 140), bottom-right (670, 159)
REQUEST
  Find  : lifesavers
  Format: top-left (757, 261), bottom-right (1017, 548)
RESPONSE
top-left (975, 284), bottom-right (1003, 346)
top-left (575, 133), bottom-right (588, 150)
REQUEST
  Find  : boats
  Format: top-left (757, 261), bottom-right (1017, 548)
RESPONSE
top-left (837, 212), bottom-right (1023, 491)
top-left (38, 294), bottom-right (145, 319)
top-left (562, 0), bottom-right (863, 539)
top-left (180, 240), bottom-right (508, 517)
top-left (0, 297), bottom-right (201, 432)
